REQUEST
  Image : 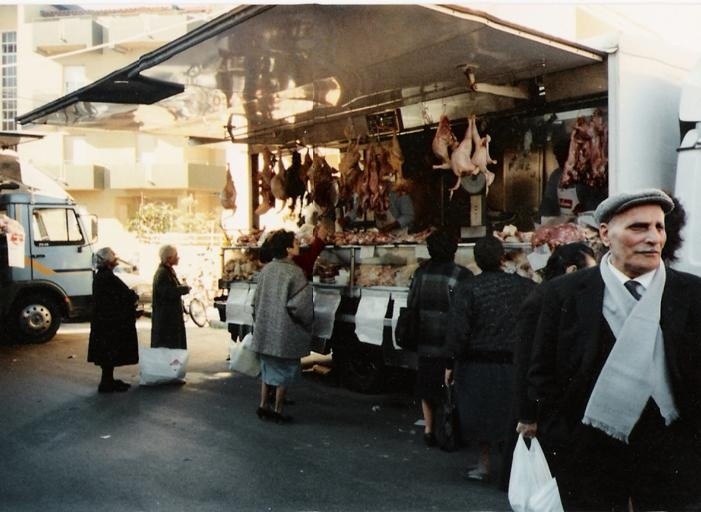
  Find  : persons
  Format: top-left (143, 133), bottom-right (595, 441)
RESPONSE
top-left (343, 191), bottom-right (417, 235)
top-left (539, 138), bottom-right (602, 219)
top-left (151, 244), bottom-right (192, 385)
top-left (259, 225), bottom-right (328, 406)
top-left (87, 247), bottom-right (139, 393)
top-left (249, 228), bottom-right (314, 424)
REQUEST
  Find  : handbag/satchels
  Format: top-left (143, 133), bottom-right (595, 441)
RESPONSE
top-left (395, 308), bottom-right (419, 353)
top-left (138, 347), bottom-right (191, 386)
top-left (229, 332), bottom-right (261, 378)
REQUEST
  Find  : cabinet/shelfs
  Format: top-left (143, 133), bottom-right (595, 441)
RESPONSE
top-left (217, 237), bottom-right (534, 292)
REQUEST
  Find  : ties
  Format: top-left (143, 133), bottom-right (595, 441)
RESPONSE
top-left (624, 281), bottom-right (641, 301)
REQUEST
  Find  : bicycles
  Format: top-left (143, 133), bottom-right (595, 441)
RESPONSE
top-left (181, 271), bottom-right (211, 330)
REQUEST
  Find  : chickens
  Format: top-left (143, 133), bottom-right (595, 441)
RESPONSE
top-left (472, 115), bottom-right (497, 198)
top-left (432, 116), bottom-right (480, 200)
top-left (254, 130), bottom-right (406, 216)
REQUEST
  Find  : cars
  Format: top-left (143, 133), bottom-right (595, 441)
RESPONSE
top-left (61, 246), bottom-right (153, 319)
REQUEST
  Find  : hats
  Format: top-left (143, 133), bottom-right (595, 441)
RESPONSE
top-left (594, 189), bottom-right (675, 224)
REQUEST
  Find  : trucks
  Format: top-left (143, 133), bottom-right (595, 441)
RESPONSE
top-left (7, 4), bottom-right (701, 395)
top-left (0, 147), bottom-right (101, 349)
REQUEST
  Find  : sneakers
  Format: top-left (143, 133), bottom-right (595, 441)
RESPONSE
top-left (98, 380), bottom-right (131, 393)
top-left (463, 464), bottom-right (488, 481)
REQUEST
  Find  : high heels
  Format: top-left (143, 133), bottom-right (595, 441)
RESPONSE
top-left (256, 407), bottom-right (292, 424)
